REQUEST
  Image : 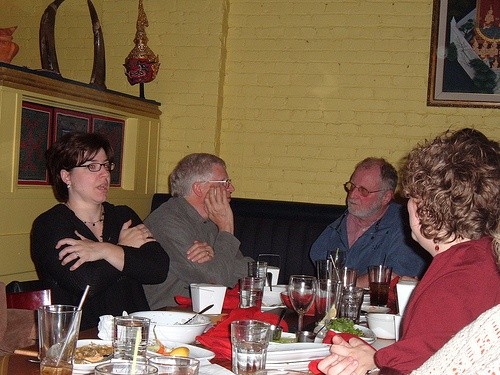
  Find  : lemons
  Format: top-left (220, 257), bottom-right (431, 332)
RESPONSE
top-left (170, 347), bottom-right (190, 357)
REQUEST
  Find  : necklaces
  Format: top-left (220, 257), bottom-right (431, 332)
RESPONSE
top-left (78, 213), bottom-right (105, 226)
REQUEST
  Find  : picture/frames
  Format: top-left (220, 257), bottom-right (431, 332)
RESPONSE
top-left (18, 101), bottom-right (125, 188)
top-left (427, 0), bottom-right (500, 109)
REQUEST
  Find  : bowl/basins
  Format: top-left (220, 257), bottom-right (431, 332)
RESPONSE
top-left (261, 285), bottom-right (287, 307)
top-left (280, 288), bottom-right (334, 317)
top-left (130, 310), bottom-right (211, 344)
top-left (359, 305), bottom-right (391, 323)
top-left (365, 313), bottom-right (397, 341)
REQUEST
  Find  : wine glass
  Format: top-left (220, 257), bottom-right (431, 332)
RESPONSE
top-left (289, 275), bottom-right (319, 342)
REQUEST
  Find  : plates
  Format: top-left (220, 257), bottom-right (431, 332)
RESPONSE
top-left (265, 344), bottom-right (333, 366)
top-left (314, 325), bottom-right (376, 346)
top-left (49, 339), bottom-right (216, 370)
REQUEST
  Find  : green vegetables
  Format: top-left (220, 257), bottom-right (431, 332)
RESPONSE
top-left (326, 317), bottom-right (365, 337)
top-left (272, 338), bottom-right (298, 343)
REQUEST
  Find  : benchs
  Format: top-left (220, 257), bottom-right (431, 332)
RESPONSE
top-left (150, 193), bottom-right (347, 285)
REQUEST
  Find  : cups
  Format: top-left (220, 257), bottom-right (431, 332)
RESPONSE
top-left (259, 253), bottom-right (281, 287)
top-left (112, 316), bottom-right (150, 363)
top-left (191, 282), bottom-right (227, 315)
top-left (313, 249), bottom-right (393, 325)
top-left (94, 361), bottom-right (158, 375)
top-left (37, 304), bottom-right (82, 375)
top-left (230, 319), bottom-right (271, 375)
top-left (238, 261), bottom-right (267, 309)
top-left (147, 355), bottom-right (200, 375)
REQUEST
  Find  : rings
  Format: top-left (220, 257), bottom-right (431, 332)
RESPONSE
top-left (206, 251), bottom-right (209, 256)
top-left (74, 250), bottom-right (80, 258)
top-left (145, 233), bottom-right (148, 237)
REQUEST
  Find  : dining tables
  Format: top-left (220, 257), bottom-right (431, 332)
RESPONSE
top-left (5, 284), bottom-right (397, 375)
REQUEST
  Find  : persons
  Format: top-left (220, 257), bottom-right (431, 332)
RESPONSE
top-left (318, 128), bottom-right (500, 375)
top-left (29, 130), bottom-right (171, 332)
top-left (140, 153), bottom-right (255, 312)
top-left (311, 157), bottom-right (423, 289)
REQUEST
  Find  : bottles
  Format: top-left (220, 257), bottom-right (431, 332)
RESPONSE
top-left (0, 25), bottom-right (20, 64)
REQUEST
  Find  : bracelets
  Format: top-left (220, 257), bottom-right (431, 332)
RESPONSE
top-left (366, 368), bottom-right (381, 375)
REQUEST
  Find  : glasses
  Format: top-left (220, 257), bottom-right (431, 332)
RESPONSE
top-left (72, 162), bottom-right (116, 172)
top-left (344, 181), bottom-right (393, 197)
top-left (195, 179), bottom-right (231, 189)
